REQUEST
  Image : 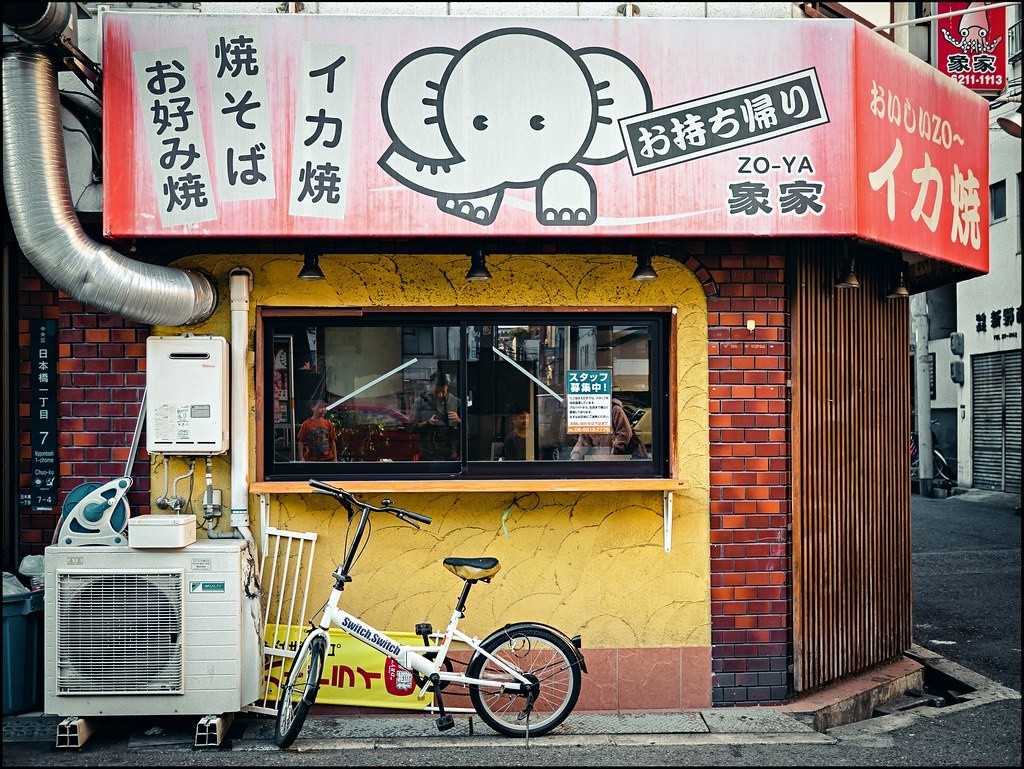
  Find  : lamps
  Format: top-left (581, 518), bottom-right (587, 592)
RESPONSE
top-left (464, 251), bottom-right (492, 281)
top-left (747, 320), bottom-right (755, 335)
top-left (834, 255), bottom-right (860, 288)
top-left (298, 252), bottom-right (325, 279)
top-left (629, 249), bottom-right (658, 280)
top-left (887, 271), bottom-right (909, 298)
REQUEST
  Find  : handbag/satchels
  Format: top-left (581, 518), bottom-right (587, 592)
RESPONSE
top-left (621, 435), bottom-right (648, 459)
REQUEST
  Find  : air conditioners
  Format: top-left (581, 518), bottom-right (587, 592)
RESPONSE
top-left (44, 540), bottom-right (263, 716)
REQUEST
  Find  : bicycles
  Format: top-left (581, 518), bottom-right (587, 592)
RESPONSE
top-left (274, 479), bottom-right (589, 752)
top-left (910, 418), bottom-right (948, 479)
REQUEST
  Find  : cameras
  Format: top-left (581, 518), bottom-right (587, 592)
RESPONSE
top-left (436, 414), bottom-right (448, 421)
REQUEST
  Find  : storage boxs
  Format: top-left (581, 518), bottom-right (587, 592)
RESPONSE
top-left (129, 514), bottom-right (197, 548)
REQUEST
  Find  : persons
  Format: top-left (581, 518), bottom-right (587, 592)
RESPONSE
top-left (504, 410), bottom-right (542, 460)
top-left (293, 355), bottom-right (325, 432)
top-left (559, 398), bottom-right (579, 460)
top-left (407, 371), bottom-right (462, 461)
top-left (297, 396), bottom-right (337, 462)
top-left (570, 398), bottom-right (648, 460)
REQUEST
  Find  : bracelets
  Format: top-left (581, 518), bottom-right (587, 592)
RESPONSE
top-left (426, 420), bottom-right (430, 425)
